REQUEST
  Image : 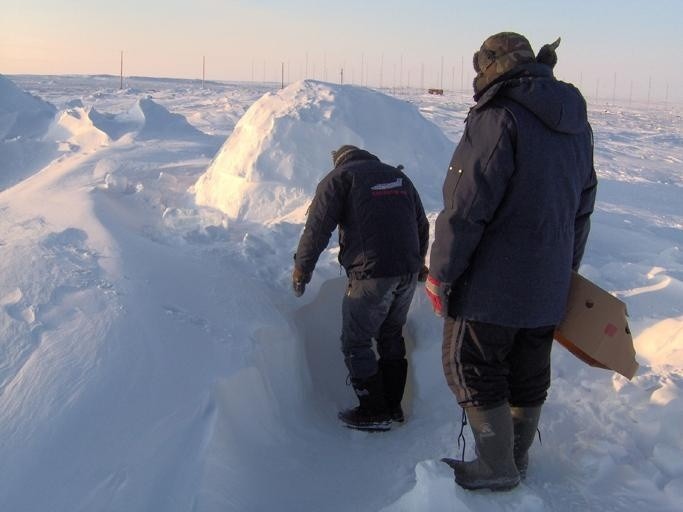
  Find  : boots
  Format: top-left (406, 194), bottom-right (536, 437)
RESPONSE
top-left (440, 398), bottom-right (543, 495)
top-left (336, 359), bottom-right (407, 432)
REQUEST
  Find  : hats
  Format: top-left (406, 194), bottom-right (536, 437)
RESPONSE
top-left (334, 145), bottom-right (360, 169)
top-left (470, 32), bottom-right (537, 95)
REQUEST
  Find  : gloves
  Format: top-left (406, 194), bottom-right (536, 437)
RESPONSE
top-left (293, 265), bottom-right (313, 297)
top-left (425, 274), bottom-right (453, 320)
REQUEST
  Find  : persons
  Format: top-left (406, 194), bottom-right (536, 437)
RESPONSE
top-left (423, 29), bottom-right (599, 494)
top-left (290, 143), bottom-right (430, 433)
